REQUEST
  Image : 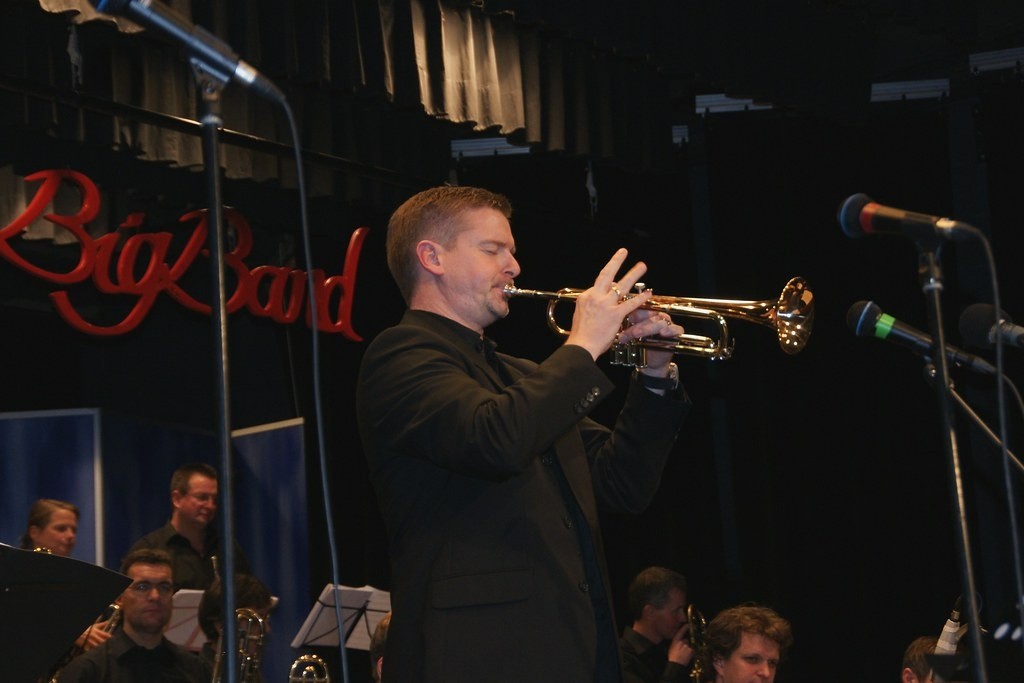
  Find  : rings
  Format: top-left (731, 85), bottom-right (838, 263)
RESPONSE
top-left (612, 287), bottom-right (622, 301)
top-left (660, 318), bottom-right (671, 326)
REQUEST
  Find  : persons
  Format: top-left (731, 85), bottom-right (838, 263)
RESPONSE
top-left (55, 547), bottom-right (214, 683)
top-left (701, 600), bottom-right (795, 683)
top-left (126, 462), bottom-right (253, 596)
top-left (197, 573), bottom-right (274, 683)
top-left (19, 498), bottom-right (79, 556)
top-left (355, 183), bottom-right (687, 683)
top-left (619, 564), bottom-right (695, 683)
top-left (902, 636), bottom-right (973, 683)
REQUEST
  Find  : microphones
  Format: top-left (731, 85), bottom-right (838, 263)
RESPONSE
top-left (844, 301), bottom-right (997, 377)
top-left (960, 303), bottom-right (1024, 352)
top-left (924, 608), bottom-right (961, 683)
top-left (833, 193), bottom-right (970, 243)
top-left (87, 0), bottom-right (276, 107)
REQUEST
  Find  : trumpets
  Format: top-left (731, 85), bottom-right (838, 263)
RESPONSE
top-left (503, 276), bottom-right (817, 370)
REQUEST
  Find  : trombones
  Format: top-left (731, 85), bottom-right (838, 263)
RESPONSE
top-left (685, 605), bottom-right (710, 683)
top-left (48, 556), bottom-right (332, 683)
